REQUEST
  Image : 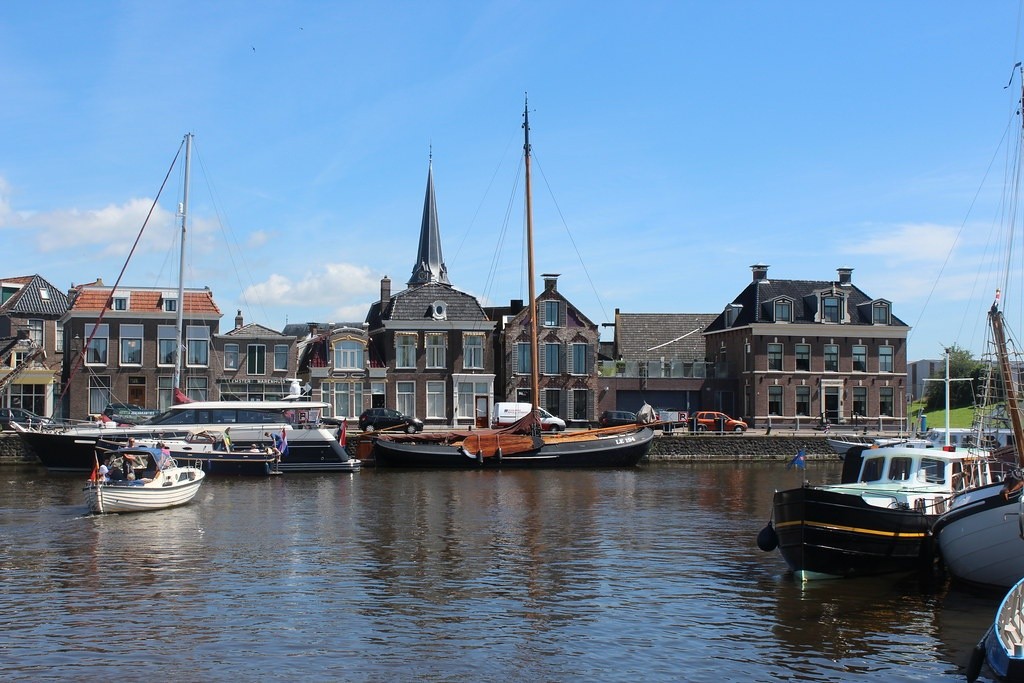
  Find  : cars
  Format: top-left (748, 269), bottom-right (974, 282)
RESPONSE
top-left (103, 403), bottom-right (143, 421)
top-left (0, 408), bottom-right (54, 432)
top-left (598, 411), bottom-right (638, 428)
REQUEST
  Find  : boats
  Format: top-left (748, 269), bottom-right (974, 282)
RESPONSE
top-left (80, 448), bottom-right (207, 516)
top-left (964, 575), bottom-right (1024, 682)
top-left (825, 427), bottom-right (1018, 466)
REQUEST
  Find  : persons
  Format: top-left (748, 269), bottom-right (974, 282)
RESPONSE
top-left (251, 443), bottom-right (273, 460)
top-left (993, 474), bottom-right (1006, 483)
top-left (265, 433), bottom-right (283, 462)
top-left (99, 437), bottom-right (171, 482)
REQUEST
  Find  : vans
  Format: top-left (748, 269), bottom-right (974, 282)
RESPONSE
top-left (491, 401), bottom-right (566, 434)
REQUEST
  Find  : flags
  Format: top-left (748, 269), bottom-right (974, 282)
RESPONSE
top-left (279, 428), bottom-right (290, 455)
top-left (89, 454), bottom-right (100, 481)
top-left (334, 419), bottom-right (347, 451)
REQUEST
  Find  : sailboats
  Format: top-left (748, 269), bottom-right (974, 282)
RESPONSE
top-left (370, 88), bottom-right (653, 468)
top-left (0, 128), bottom-right (367, 474)
top-left (757, 350), bottom-right (1001, 580)
top-left (929, 289), bottom-right (1024, 595)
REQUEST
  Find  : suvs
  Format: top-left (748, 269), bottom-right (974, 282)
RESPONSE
top-left (687, 411), bottom-right (748, 434)
top-left (358, 408), bottom-right (426, 435)
